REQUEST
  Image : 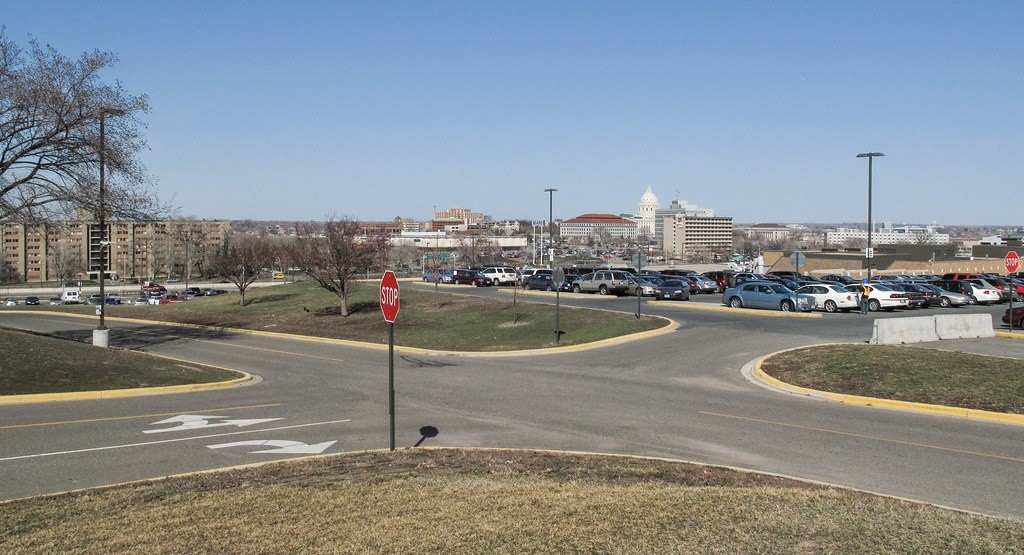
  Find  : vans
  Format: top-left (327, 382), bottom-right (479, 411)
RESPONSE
top-left (61, 291), bottom-right (79, 304)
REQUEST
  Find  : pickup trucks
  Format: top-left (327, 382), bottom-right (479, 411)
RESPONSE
top-left (105, 293), bottom-right (121, 302)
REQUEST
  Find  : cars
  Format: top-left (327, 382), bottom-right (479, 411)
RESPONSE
top-left (128, 281), bottom-right (229, 305)
top-left (1002, 306), bottom-right (1024, 327)
top-left (1, 297), bottom-right (18, 306)
top-left (49, 297), bottom-right (63, 306)
top-left (423, 263), bottom-right (1024, 311)
top-left (64, 282), bottom-right (77, 287)
top-left (104, 298), bottom-right (117, 305)
top-left (24, 296), bottom-right (40, 305)
top-left (274, 273), bottom-right (285, 280)
top-left (80, 294), bottom-right (100, 304)
top-left (722, 281), bottom-right (818, 313)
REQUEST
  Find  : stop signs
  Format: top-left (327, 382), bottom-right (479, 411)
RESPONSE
top-left (378, 271), bottom-right (400, 324)
top-left (1005, 251), bottom-right (1020, 271)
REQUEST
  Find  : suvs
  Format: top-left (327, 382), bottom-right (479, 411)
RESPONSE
top-left (573, 270), bottom-right (629, 296)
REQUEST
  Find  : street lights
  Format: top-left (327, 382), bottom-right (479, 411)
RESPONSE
top-left (636, 245), bottom-right (641, 319)
top-left (132, 223), bottom-right (140, 281)
top-left (545, 188), bottom-right (558, 268)
top-left (97, 108), bottom-right (126, 327)
top-left (858, 152), bottom-right (883, 281)
